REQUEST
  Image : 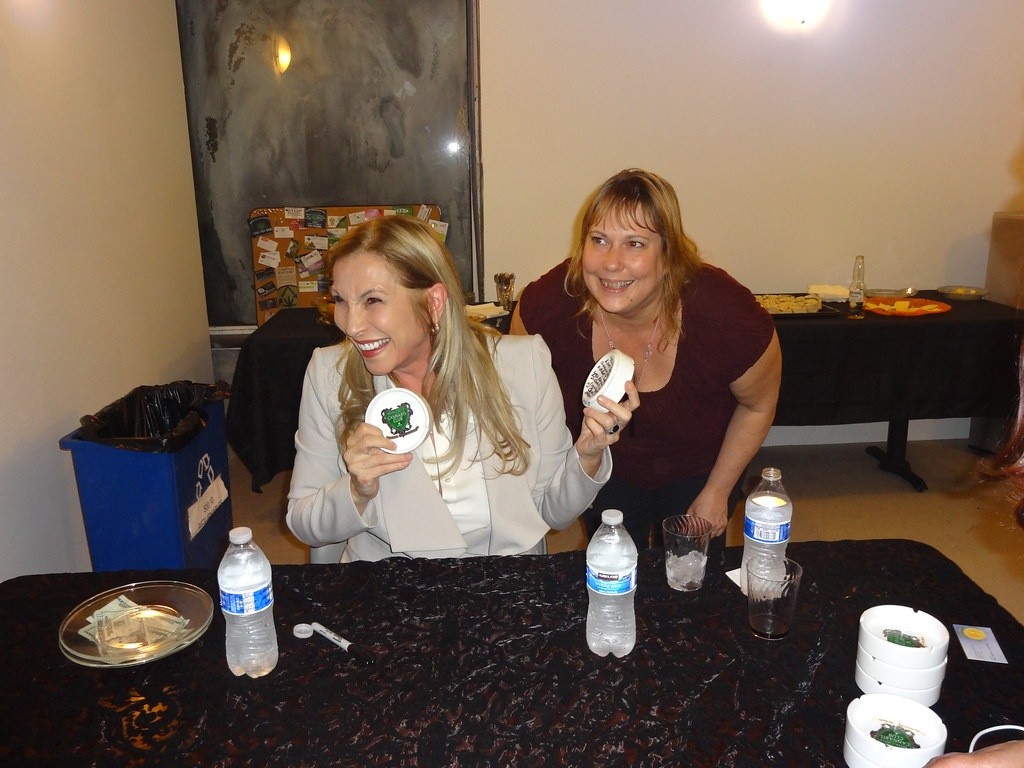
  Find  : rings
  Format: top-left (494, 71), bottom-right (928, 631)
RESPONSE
top-left (608, 423), bottom-right (620, 436)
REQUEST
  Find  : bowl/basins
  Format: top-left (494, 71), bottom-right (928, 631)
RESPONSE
top-left (312, 295), bottom-right (335, 323)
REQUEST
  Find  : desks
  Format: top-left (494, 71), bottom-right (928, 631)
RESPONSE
top-left (225, 290), bottom-right (1024, 493)
top-left (0, 537), bottom-right (1024, 768)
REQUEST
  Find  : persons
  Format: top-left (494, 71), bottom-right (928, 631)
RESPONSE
top-left (284, 213), bottom-right (641, 564)
top-left (506, 167), bottom-right (783, 553)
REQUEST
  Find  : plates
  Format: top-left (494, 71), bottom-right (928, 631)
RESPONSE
top-left (58, 580), bottom-right (214, 667)
top-left (866, 289), bottom-right (918, 298)
top-left (486, 310), bottom-right (510, 318)
top-left (937, 286), bottom-right (991, 300)
top-left (865, 298), bottom-right (951, 316)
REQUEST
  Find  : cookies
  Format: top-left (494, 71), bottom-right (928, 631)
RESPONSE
top-left (865, 300), bottom-right (940, 311)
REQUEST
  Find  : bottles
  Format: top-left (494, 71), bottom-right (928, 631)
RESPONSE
top-left (740, 468), bottom-right (793, 597)
top-left (848, 256), bottom-right (865, 319)
top-left (586, 509), bottom-right (637, 658)
top-left (217, 527), bottom-right (279, 678)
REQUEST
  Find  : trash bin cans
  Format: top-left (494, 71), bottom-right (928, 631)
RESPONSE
top-left (58, 379), bottom-right (234, 571)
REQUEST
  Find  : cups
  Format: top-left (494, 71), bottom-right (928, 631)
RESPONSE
top-left (746, 555), bottom-right (803, 641)
top-left (496, 285), bottom-right (514, 310)
top-left (662, 515), bottom-right (713, 592)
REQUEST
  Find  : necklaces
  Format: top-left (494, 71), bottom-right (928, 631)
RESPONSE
top-left (596, 303), bottom-right (662, 389)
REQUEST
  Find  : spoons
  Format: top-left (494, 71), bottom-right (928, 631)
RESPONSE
top-left (494, 273), bottom-right (516, 308)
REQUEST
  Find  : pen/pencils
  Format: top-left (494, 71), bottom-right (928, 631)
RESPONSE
top-left (311, 621), bottom-right (374, 665)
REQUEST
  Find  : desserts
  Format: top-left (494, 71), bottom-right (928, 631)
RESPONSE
top-left (752, 294), bottom-right (822, 314)
